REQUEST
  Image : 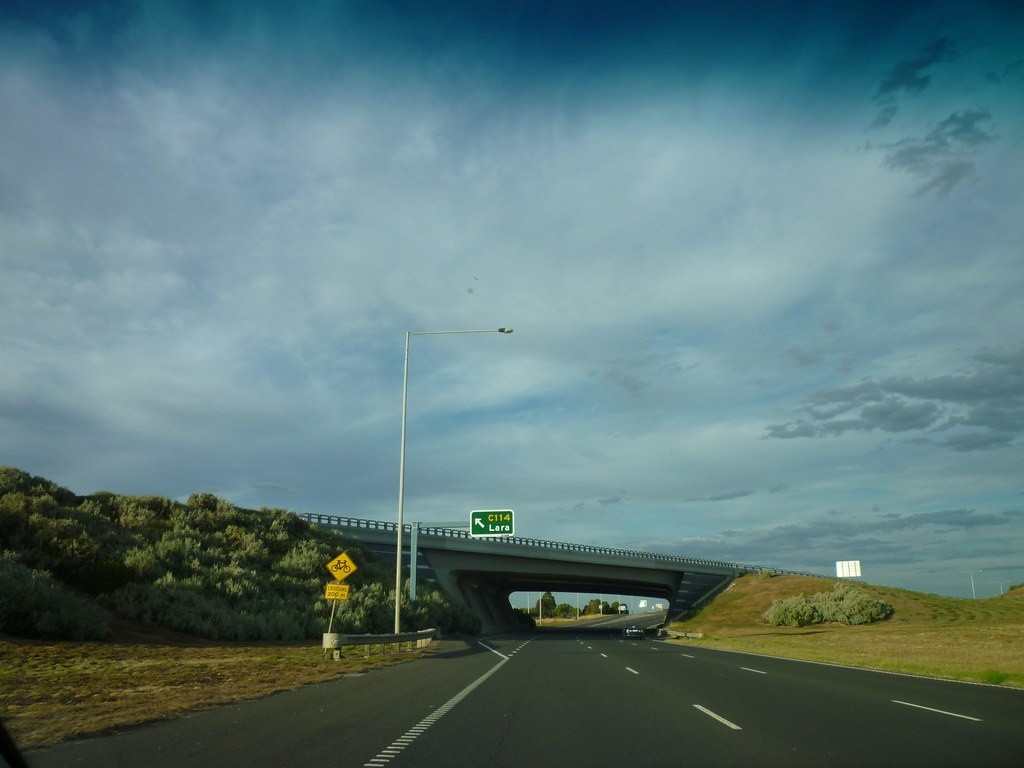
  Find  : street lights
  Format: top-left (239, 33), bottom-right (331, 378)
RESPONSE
top-left (392, 327), bottom-right (515, 638)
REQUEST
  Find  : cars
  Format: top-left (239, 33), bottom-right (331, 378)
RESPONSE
top-left (623, 625), bottom-right (645, 640)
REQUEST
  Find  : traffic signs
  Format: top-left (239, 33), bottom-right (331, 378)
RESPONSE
top-left (469, 509), bottom-right (514, 537)
